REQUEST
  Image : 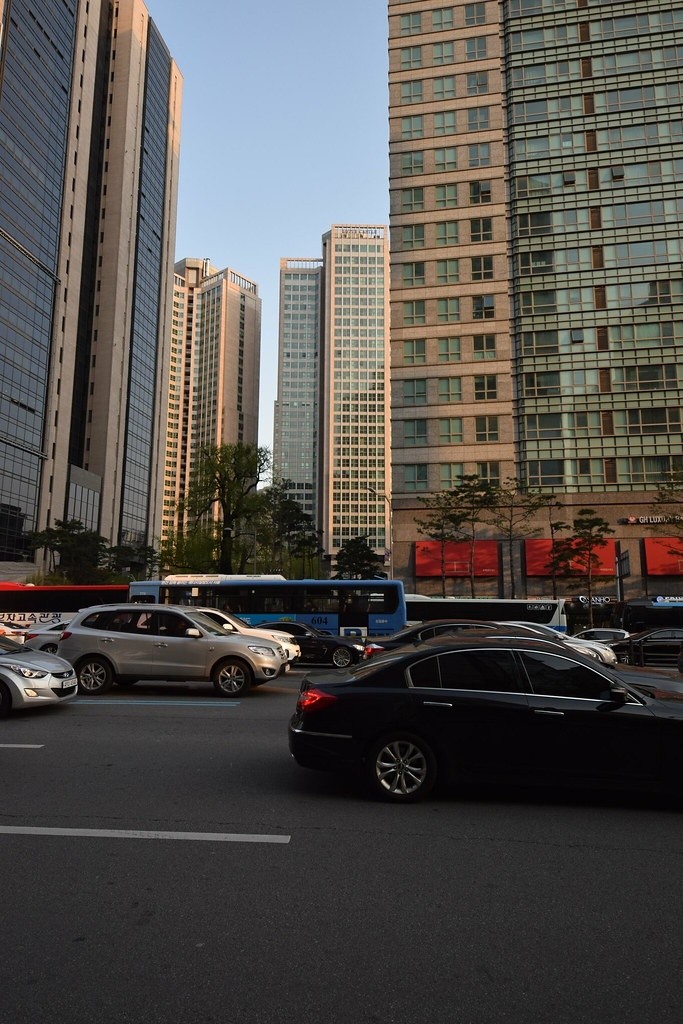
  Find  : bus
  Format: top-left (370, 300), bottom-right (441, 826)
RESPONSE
top-left (613, 600), bottom-right (683, 633)
top-left (367, 592), bottom-right (568, 635)
top-left (160, 573), bottom-right (406, 639)
top-left (129, 582), bottom-right (193, 606)
top-left (0, 580), bottom-right (130, 628)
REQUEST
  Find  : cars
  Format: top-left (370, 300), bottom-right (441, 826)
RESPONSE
top-left (24, 619), bottom-right (73, 655)
top-left (254, 622), bottom-right (368, 668)
top-left (358, 618), bottom-right (509, 664)
top-left (494, 620), bottom-right (617, 666)
top-left (288, 633), bottom-right (683, 803)
top-left (0, 635), bottom-right (78, 714)
top-left (570, 627), bottom-right (631, 643)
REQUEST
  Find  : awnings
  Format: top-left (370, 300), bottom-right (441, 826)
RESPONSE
top-left (415, 541), bottom-right (500, 577)
top-left (525, 539), bottom-right (616, 576)
top-left (643, 537), bottom-right (683, 576)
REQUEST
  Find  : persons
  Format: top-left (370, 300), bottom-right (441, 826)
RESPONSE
top-left (304, 600), bottom-right (318, 611)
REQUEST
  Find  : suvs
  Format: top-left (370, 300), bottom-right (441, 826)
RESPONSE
top-left (129, 605), bottom-right (301, 676)
top-left (57, 604), bottom-right (287, 697)
top-left (603, 628), bottom-right (683, 668)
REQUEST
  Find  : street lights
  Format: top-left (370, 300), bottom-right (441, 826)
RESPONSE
top-left (225, 528), bottom-right (257, 575)
top-left (367, 487), bottom-right (393, 580)
